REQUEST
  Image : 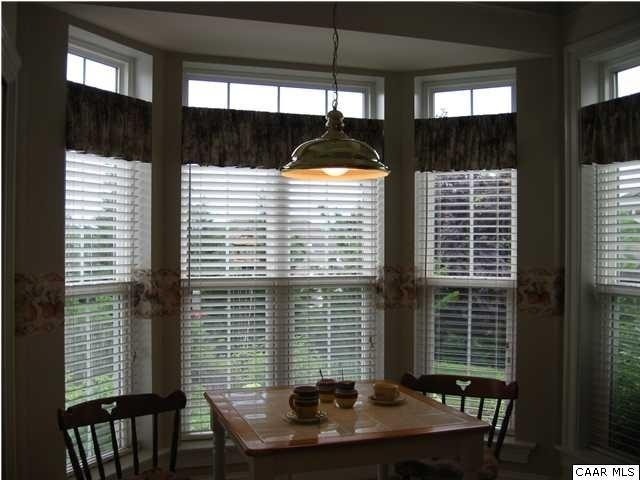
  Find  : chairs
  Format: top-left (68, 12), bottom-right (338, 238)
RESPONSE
top-left (394, 373), bottom-right (518, 478)
top-left (57, 390), bottom-right (187, 479)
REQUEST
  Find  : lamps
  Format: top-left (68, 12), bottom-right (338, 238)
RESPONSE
top-left (279, 1), bottom-right (393, 180)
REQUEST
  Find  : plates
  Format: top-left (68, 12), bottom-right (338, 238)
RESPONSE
top-left (286, 410), bottom-right (328, 422)
top-left (369, 394), bottom-right (408, 404)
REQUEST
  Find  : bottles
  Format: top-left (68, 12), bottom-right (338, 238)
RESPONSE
top-left (335, 380), bottom-right (359, 409)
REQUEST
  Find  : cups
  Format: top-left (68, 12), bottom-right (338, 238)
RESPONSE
top-left (374, 383), bottom-right (400, 400)
top-left (316, 379), bottom-right (335, 404)
top-left (288, 386), bottom-right (319, 417)
